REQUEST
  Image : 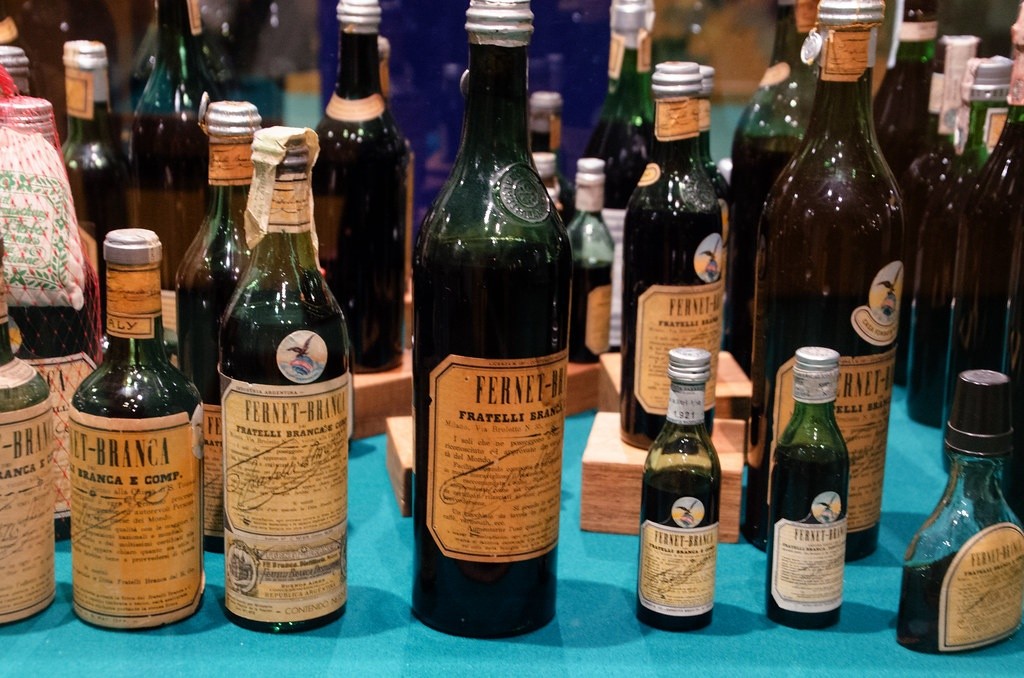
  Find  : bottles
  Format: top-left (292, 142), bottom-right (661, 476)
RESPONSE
top-left (0, 0), bottom-right (1024, 654)
